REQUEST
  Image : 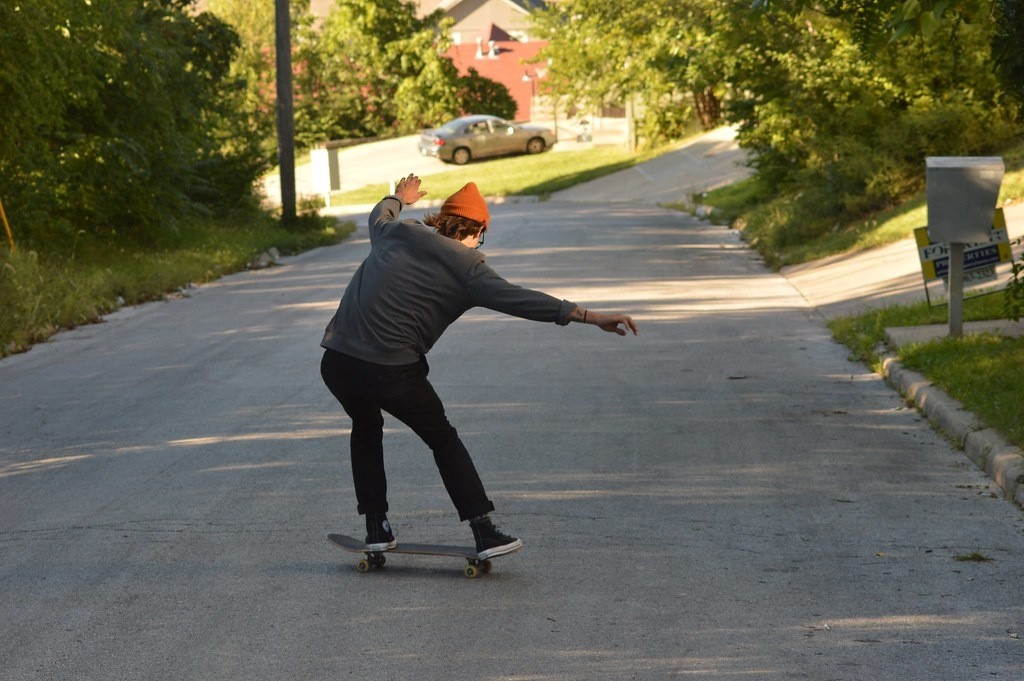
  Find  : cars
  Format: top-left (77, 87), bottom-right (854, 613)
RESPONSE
top-left (420, 114), bottom-right (558, 166)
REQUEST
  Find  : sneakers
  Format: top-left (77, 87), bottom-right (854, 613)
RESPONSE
top-left (469, 516), bottom-right (522, 560)
top-left (366, 512), bottom-right (396, 552)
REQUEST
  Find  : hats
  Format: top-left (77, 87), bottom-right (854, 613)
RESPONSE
top-left (441, 182), bottom-right (489, 230)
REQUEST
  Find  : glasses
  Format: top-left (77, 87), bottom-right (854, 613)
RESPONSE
top-left (475, 229), bottom-right (484, 249)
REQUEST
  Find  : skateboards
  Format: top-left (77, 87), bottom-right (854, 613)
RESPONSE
top-left (324, 531), bottom-right (507, 577)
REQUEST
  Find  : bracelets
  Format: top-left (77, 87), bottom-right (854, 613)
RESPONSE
top-left (584, 310), bottom-right (587, 323)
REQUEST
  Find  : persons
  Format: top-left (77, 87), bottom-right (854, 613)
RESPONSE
top-left (321, 171), bottom-right (638, 558)
